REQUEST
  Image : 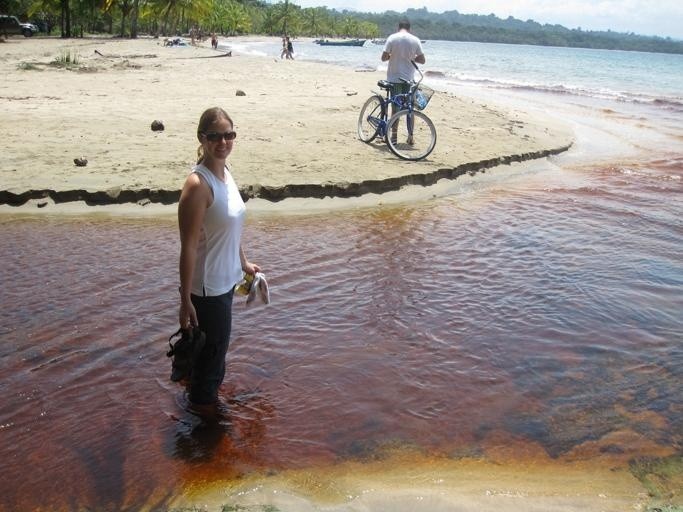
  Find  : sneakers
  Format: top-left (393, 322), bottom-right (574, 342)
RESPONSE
top-left (390, 137), bottom-right (397, 144)
top-left (406, 139), bottom-right (415, 145)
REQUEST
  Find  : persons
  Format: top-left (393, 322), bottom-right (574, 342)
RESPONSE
top-left (285, 35), bottom-right (295, 60)
top-left (280, 37), bottom-right (288, 60)
top-left (381, 18), bottom-right (426, 148)
top-left (175, 104), bottom-right (263, 404)
top-left (210, 30), bottom-right (217, 47)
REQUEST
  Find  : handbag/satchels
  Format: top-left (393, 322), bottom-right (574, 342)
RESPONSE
top-left (168, 321), bottom-right (219, 387)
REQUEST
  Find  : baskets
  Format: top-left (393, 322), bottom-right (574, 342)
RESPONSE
top-left (414, 83), bottom-right (434, 110)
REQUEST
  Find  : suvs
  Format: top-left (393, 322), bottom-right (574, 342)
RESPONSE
top-left (0, 14), bottom-right (40, 37)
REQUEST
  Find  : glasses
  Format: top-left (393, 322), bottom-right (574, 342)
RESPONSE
top-left (199, 129), bottom-right (237, 142)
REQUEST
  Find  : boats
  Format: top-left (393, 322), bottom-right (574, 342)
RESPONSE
top-left (312, 39), bottom-right (427, 46)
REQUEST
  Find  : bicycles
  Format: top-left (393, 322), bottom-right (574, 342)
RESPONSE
top-left (355, 58), bottom-right (440, 163)
